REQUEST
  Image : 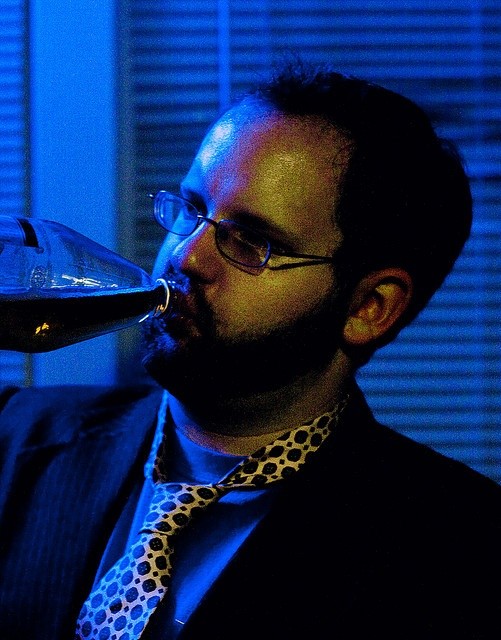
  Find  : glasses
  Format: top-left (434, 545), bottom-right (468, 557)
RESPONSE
top-left (139, 181), bottom-right (337, 272)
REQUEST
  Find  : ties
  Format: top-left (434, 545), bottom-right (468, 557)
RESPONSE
top-left (72, 384), bottom-right (351, 638)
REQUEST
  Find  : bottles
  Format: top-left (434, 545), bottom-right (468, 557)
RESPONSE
top-left (0, 217), bottom-right (186, 354)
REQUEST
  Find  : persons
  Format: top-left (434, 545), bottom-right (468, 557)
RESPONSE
top-left (2, 56), bottom-right (499, 637)
